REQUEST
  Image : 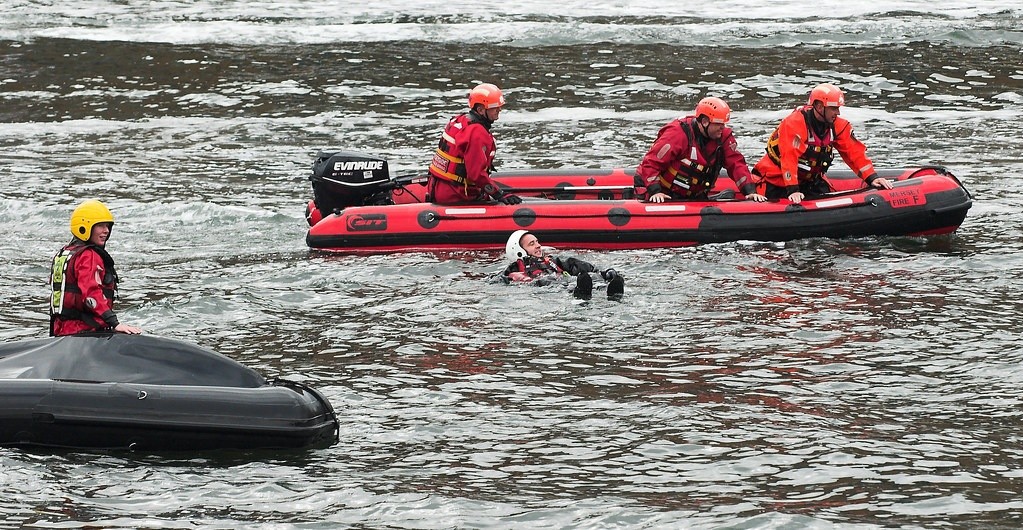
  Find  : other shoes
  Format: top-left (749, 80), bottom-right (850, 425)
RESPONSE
top-left (707, 188), bottom-right (735, 201)
top-left (606, 277), bottom-right (624, 302)
top-left (573, 272), bottom-right (593, 305)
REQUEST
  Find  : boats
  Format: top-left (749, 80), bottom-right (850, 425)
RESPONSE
top-left (0, 333), bottom-right (341, 450)
top-left (306, 146), bottom-right (975, 253)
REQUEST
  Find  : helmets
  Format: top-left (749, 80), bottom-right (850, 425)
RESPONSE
top-left (695, 97), bottom-right (731, 124)
top-left (807, 83), bottom-right (845, 107)
top-left (468, 83), bottom-right (505, 110)
top-left (506, 230), bottom-right (530, 263)
top-left (70, 200), bottom-right (115, 241)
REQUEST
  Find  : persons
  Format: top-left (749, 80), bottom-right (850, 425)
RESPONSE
top-left (634, 98), bottom-right (768, 203)
top-left (751, 83), bottom-right (894, 203)
top-left (49, 200), bottom-right (141, 336)
top-left (503, 230), bottom-right (624, 298)
top-left (429, 83), bottom-right (522, 205)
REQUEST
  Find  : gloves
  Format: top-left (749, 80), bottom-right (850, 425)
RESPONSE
top-left (499, 192), bottom-right (523, 206)
